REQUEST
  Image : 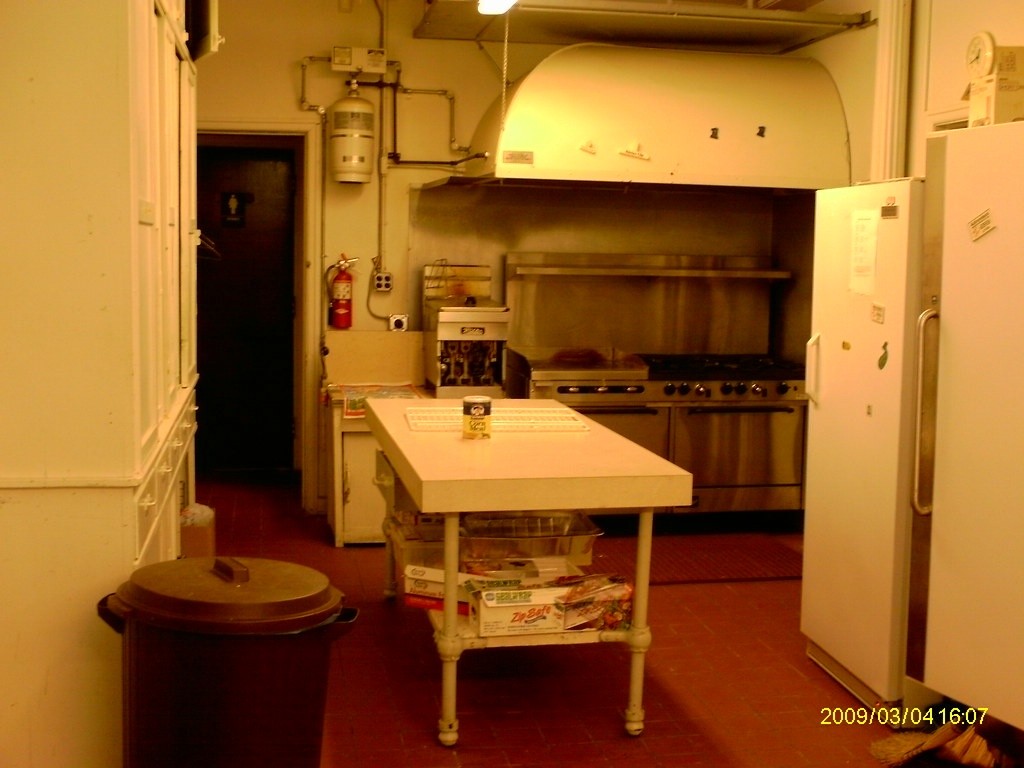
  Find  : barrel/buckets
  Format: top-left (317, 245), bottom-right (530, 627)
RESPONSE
top-left (97, 555), bottom-right (362, 767)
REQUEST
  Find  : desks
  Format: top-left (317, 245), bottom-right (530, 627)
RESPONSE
top-left (365, 398), bottom-right (693, 745)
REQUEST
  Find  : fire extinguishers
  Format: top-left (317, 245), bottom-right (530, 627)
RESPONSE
top-left (325, 256), bottom-right (357, 329)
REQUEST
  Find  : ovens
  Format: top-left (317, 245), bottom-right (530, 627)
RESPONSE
top-left (531, 401), bottom-right (806, 513)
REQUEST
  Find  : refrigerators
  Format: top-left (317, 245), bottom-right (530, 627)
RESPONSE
top-left (800, 175), bottom-right (930, 730)
top-left (891, 118), bottom-right (1023, 738)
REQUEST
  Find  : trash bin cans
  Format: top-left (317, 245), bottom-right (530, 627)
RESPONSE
top-left (94, 550), bottom-right (361, 767)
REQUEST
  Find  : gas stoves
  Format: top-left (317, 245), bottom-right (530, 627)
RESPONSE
top-left (640, 353), bottom-right (806, 405)
